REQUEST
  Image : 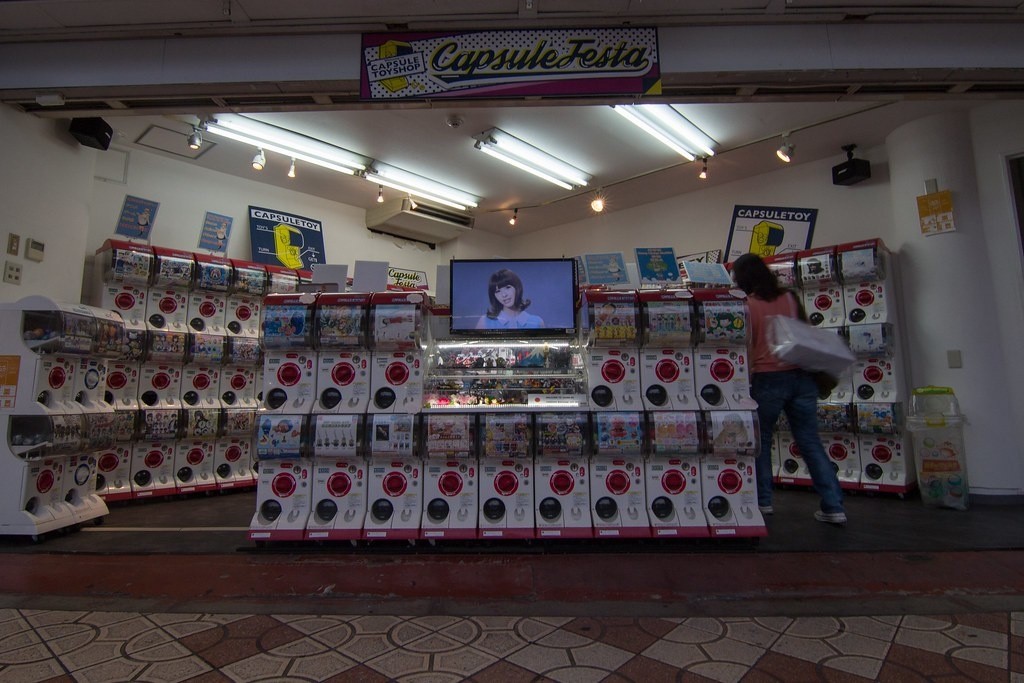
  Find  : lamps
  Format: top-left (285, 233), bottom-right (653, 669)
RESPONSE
top-left (595, 191), bottom-right (603, 211)
top-left (473, 128), bottom-right (595, 191)
top-left (777, 130), bottom-right (796, 163)
top-left (407, 192), bottom-right (416, 210)
top-left (198, 113), bottom-right (373, 178)
top-left (700, 157), bottom-right (708, 179)
top-left (253, 147), bottom-right (266, 170)
top-left (186, 125), bottom-right (204, 150)
top-left (512, 207), bottom-right (518, 225)
top-left (289, 156), bottom-right (295, 178)
top-left (362, 160), bottom-right (486, 214)
top-left (609, 104), bottom-right (718, 162)
top-left (378, 185), bottom-right (384, 202)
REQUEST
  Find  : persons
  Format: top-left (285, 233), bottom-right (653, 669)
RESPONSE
top-left (476, 269), bottom-right (545, 329)
top-left (730, 252), bottom-right (847, 523)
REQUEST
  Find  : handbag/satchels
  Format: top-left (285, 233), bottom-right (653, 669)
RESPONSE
top-left (793, 291), bottom-right (838, 400)
top-left (769, 315), bottom-right (856, 375)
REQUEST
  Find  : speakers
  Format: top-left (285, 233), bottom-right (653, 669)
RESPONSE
top-left (832, 157), bottom-right (871, 186)
top-left (68, 117), bottom-right (114, 151)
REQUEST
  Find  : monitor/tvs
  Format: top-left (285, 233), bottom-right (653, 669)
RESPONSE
top-left (449, 257), bottom-right (576, 336)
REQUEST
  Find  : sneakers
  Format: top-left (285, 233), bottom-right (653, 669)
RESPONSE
top-left (814, 509), bottom-right (846, 523)
top-left (759, 505), bottom-right (772, 513)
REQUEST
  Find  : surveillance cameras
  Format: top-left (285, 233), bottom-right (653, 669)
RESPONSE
top-left (446, 117), bottom-right (464, 129)
